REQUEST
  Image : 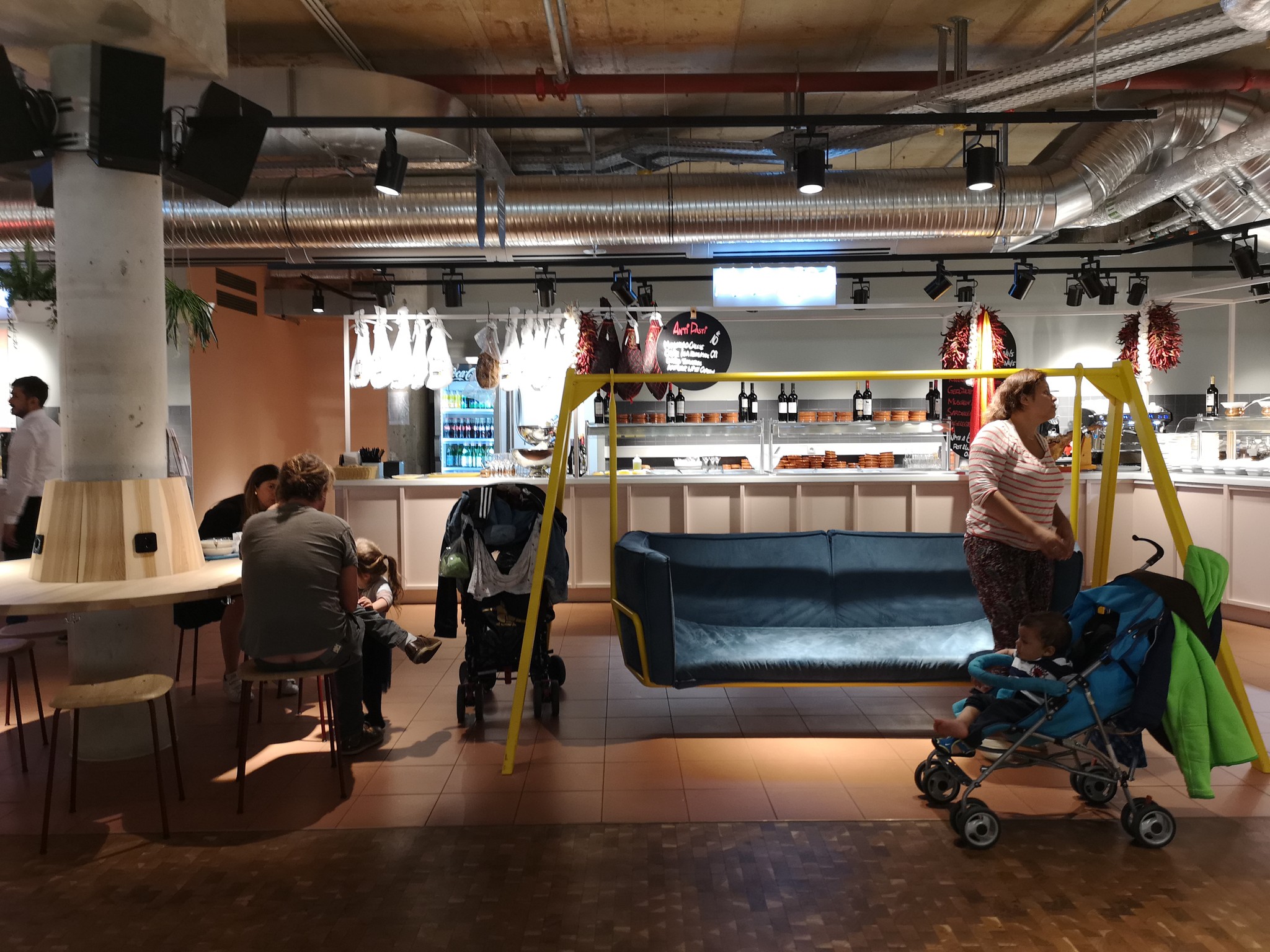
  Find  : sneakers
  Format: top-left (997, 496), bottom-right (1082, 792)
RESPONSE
top-left (223, 671), bottom-right (254, 703)
top-left (272, 677), bottom-right (299, 695)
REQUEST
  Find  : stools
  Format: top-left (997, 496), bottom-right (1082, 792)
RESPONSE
top-left (0, 619), bottom-right (338, 855)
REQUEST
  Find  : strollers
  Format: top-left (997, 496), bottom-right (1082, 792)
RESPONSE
top-left (913, 534), bottom-right (1224, 850)
top-left (446, 481), bottom-right (566, 726)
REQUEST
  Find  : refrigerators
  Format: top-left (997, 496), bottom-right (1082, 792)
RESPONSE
top-left (433, 356), bottom-right (524, 474)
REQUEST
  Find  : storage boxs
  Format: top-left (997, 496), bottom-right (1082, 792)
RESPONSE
top-left (362, 460), bottom-right (404, 479)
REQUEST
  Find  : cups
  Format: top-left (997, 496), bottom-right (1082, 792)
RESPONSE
top-left (1148, 402), bottom-right (1163, 413)
top-left (902, 451), bottom-right (942, 469)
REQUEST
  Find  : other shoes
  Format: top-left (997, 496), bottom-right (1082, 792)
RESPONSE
top-left (342, 726), bottom-right (384, 755)
top-left (404, 634), bottom-right (442, 665)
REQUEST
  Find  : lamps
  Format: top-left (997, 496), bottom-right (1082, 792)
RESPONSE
top-left (963, 124), bottom-right (999, 192)
top-left (375, 128), bottom-right (407, 195)
top-left (302, 266), bottom-right (658, 315)
top-left (792, 127), bottom-right (832, 194)
top-left (850, 257), bottom-right (1149, 305)
top-left (1230, 235), bottom-right (1270, 304)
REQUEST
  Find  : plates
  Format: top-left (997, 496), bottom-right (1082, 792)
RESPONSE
top-left (1220, 402), bottom-right (1248, 409)
top-left (392, 474), bottom-right (424, 479)
top-left (1257, 401), bottom-right (1270, 407)
top-left (201, 539), bottom-right (235, 556)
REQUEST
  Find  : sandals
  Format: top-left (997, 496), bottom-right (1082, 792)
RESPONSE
top-left (932, 735), bottom-right (976, 758)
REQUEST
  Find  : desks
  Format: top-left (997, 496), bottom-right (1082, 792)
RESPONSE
top-left (0, 477), bottom-right (243, 760)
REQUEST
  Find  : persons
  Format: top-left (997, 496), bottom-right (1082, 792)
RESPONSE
top-left (199, 464), bottom-right (299, 704)
top-left (354, 538), bottom-right (404, 730)
top-left (933, 611), bottom-right (1073, 757)
top-left (963, 369), bottom-right (1075, 651)
top-left (3, 376), bottom-right (60, 561)
top-left (237, 453), bottom-right (442, 754)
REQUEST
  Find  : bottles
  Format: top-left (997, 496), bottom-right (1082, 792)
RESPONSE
top-left (666, 382), bottom-right (685, 423)
top-left (444, 389), bottom-right (494, 409)
top-left (738, 381), bottom-right (758, 423)
top-left (446, 441), bottom-right (494, 467)
top-left (594, 387), bottom-right (610, 424)
top-left (1206, 376), bottom-right (1219, 417)
top-left (1219, 435), bottom-right (1270, 461)
top-left (925, 380), bottom-right (940, 420)
top-left (632, 454), bottom-right (642, 469)
top-left (567, 434), bottom-right (587, 477)
top-left (853, 380), bottom-right (873, 421)
top-left (778, 383), bottom-right (798, 422)
top-left (444, 417), bottom-right (493, 438)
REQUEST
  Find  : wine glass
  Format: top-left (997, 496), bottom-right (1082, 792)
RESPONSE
top-left (480, 453), bottom-right (529, 477)
top-left (702, 455), bottom-right (720, 472)
